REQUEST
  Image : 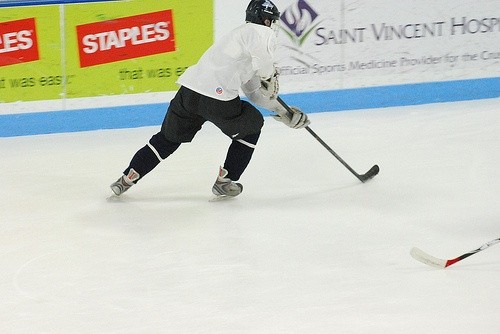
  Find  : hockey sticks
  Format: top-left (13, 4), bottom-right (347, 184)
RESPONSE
top-left (261, 80), bottom-right (380, 184)
top-left (409, 237), bottom-right (500, 269)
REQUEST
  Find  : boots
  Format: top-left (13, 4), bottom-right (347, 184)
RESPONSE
top-left (111, 169), bottom-right (141, 197)
top-left (208, 164), bottom-right (245, 203)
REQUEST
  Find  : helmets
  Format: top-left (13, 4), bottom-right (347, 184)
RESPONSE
top-left (244, 0), bottom-right (282, 28)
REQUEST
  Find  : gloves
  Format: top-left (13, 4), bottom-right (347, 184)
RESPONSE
top-left (269, 104), bottom-right (311, 131)
top-left (261, 72), bottom-right (280, 100)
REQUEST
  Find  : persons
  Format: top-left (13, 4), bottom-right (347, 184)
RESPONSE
top-left (107, 0), bottom-right (311, 203)
